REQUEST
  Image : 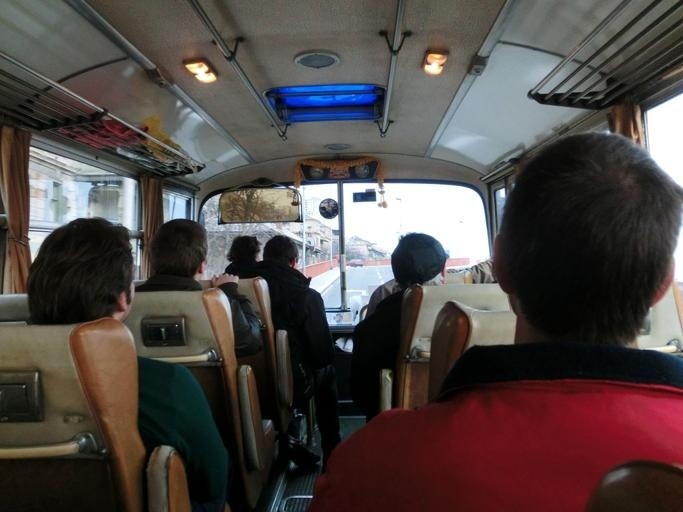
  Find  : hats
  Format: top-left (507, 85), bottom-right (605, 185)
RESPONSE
top-left (391, 233), bottom-right (450, 285)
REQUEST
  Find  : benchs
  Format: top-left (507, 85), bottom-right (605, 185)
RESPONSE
top-left (380, 272), bottom-right (682, 418)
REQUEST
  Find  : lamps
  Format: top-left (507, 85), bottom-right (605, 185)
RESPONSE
top-left (182, 58), bottom-right (221, 84)
top-left (418, 46), bottom-right (449, 76)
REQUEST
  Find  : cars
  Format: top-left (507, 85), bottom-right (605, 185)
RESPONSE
top-left (350, 259), bottom-right (364, 267)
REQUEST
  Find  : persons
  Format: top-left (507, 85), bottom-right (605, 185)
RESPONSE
top-left (224, 235), bottom-right (342, 473)
top-left (134, 220), bottom-right (263, 424)
top-left (354, 232), bottom-right (450, 421)
top-left (307, 132), bottom-right (683, 511)
top-left (25, 219), bottom-right (229, 512)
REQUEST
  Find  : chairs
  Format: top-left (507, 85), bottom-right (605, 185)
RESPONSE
top-left (0, 275), bottom-right (294, 512)
